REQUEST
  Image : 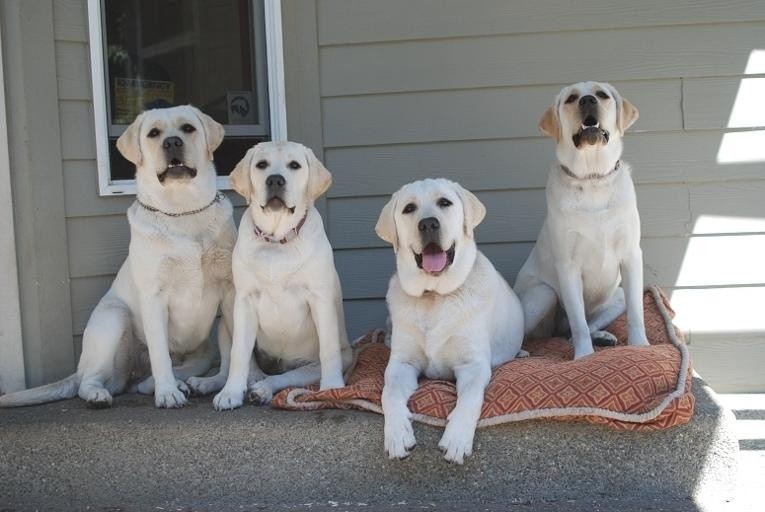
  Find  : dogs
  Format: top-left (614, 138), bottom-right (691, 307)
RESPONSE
top-left (374, 177), bottom-right (525, 467)
top-left (0, 103), bottom-right (270, 408)
top-left (184, 140), bottom-right (354, 412)
top-left (512, 80), bottom-right (652, 362)
